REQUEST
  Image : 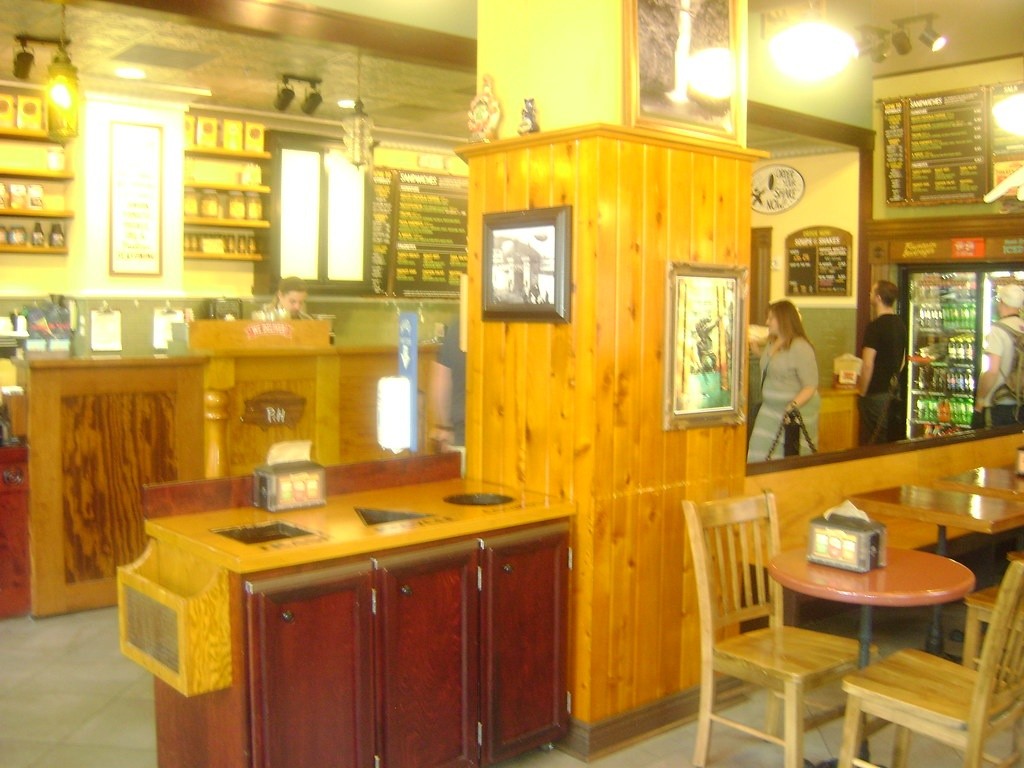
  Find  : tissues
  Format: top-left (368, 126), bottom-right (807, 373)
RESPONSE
top-left (253, 439), bottom-right (327, 512)
top-left (1013, 445), bottom-right (1024, 477)
top-left (805, 498), bottom-right (889, 574)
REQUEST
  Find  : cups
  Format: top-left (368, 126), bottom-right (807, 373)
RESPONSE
top-left (185, 189), bottom-right (262, 221)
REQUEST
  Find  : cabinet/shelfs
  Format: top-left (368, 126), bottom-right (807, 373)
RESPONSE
top-left (242, 518), bottom-right (577, 768)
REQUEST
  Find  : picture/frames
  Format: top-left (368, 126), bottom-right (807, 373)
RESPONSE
top-left (481, 203), bottom-right (574, 323)
top-left (621, 0), bottom-right (744, 149)
top-left (664, 260), bottom-right (747, 429)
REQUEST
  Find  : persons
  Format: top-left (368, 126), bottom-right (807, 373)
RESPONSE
top-left (976, 284), bottom-right (1024, 427)
top-left (747, 300), bottom-right (820, 462)
top-left (434, 323), bottom-right (466, 448)
top-left (258, 275), bottom-right (311, 319)
top-left (858, 281), bottom-right (907, 445)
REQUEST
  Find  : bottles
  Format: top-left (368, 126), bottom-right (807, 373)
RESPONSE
top-left (185, 234), bottom-right (256, 254)
top-left (32, 223), bottom-right (45, 245)
top-left (49, 225), bottom-right (63, 247)
top-left (0, 224), bottom-right (27, 244)
top-left (914, 273), bottom-right (978, 438)
top-left (0, 183), bottom-right (44, 211)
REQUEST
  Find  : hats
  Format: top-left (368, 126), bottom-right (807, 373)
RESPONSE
top-left (997, 284), bottom-right (1024, 307)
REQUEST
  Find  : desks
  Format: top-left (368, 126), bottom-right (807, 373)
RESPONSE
top-left (769, 543), bottom-right (975, 768)
top-left (846, 467), bottom-right (1024, 661)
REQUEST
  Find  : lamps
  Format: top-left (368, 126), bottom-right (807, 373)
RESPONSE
top-left (273, 71), bottom-right (324, 115)
top-left (858, 11), bottom-right (946, 63)
top-left (12, 33), bottom-right (71, 79)
top-left (341, 45), bottom-right (376, 167)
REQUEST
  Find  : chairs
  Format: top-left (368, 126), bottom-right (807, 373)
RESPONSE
top-left (682, 487), bottom-right (876, 768)
top-left (835, 554), bottom-right (1024, 768)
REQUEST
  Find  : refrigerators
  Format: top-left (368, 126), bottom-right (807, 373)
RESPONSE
top-left (898, 263), bottom-right (1024, 440)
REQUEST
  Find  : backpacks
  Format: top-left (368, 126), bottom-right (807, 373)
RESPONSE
top-left (992, 321), bottom-right (1023, 421)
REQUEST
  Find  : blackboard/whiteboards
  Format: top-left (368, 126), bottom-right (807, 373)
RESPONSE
top-left (785, 226), bottom-right (852, 297)
top-left (880, 84), bottom-right (988, 207)
top-left (371, 167), bottom-right (468, 298)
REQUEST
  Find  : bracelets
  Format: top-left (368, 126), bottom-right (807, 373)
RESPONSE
top-left (436, 425), bottom-right (455, 432)
